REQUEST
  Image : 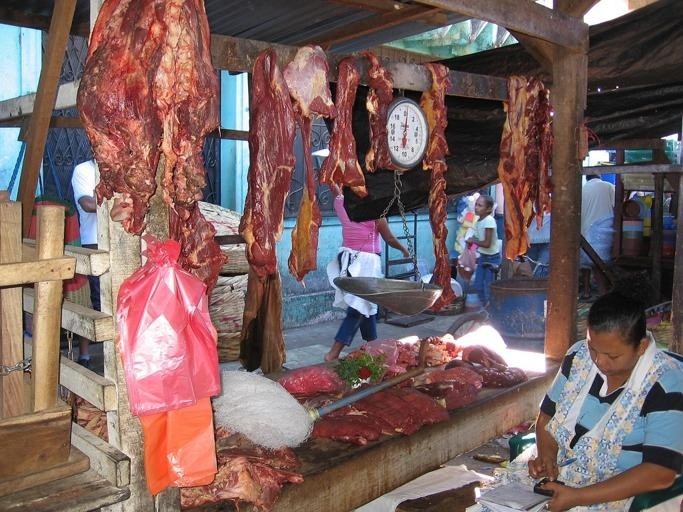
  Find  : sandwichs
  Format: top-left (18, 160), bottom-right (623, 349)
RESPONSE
top-left (212, 368), bottom-right (425, 453)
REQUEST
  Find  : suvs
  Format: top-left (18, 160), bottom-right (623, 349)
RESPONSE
top-left (489, 277), bottom-right (557, 353)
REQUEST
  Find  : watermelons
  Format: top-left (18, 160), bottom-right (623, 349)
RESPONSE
top-left (333, 89), bottom-right (444, 316)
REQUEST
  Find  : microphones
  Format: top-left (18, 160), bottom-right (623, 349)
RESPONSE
top-left (0, 189), bottom-right (89, 496)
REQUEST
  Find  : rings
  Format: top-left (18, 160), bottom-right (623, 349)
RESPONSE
top-left (527, 458), bottom-right (577, 477)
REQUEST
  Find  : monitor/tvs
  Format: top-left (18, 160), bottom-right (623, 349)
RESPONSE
top-left (475, 483), bottom-right (553, 512)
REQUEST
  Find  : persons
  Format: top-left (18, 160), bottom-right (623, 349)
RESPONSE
top-left (70, 158), bottom-right (101, 313)
top-left (465, 289), bottom-right (683, 512)
top-left (323, 192), bottom-right (411, 363)
top-left (453, 173), bottom-right (677, 312)
top-left (29, 200), bottom-right (94, 370)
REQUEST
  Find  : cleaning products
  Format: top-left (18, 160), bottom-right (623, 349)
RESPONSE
top-left (376, 209), bottom-right (436, 328)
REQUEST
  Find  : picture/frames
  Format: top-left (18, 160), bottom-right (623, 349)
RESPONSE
top-left (181, 335), bottom-right (559, 512)
